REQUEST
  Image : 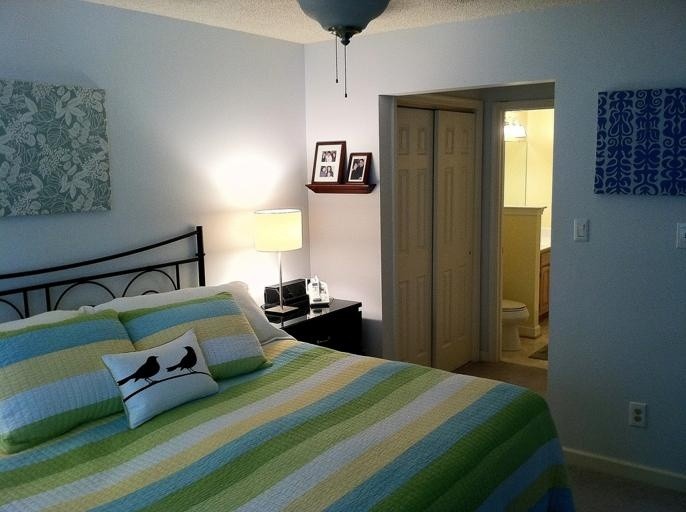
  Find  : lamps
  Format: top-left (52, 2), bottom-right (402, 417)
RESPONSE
top-left (252, 208), bottom-right (302, 315)
top-left (504, 114), bottom-right (527, 143)
top-left (296, 0), bottom-right (393, 98)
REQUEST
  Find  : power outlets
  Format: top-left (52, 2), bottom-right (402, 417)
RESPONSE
top-left (628, 400), bottom-right (649, 427)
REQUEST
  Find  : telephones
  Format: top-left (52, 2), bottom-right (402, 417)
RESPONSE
top-left (305, 275), bottom-right (330, 305)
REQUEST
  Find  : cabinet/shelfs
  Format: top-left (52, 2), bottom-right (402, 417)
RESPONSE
top-left (537, 244), bottom-right (550, 318)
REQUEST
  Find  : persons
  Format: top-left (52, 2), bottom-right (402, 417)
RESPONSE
top-left (321, 151), bottom-right (336, 161)
top-left (351, 157), bottom-right (365, 180)
top-left (320, 166), bottom-right (333, 176)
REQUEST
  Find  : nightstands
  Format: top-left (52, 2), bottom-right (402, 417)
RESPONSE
top-left (260, 294), bottom-right (363, 359)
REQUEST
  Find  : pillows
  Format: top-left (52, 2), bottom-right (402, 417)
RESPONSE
top-left (97, 326), bottom-right (220, 429)
top-left (92, 280), bottom-right (299, 346)
top-left (1, 307), bottom-right (99, 338)
top-left (1, 309), bottom-right (139, 453)
top-left (115, 289), bottom-right (273, 378)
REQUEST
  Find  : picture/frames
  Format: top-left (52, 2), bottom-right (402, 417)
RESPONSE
top-left (2, 79), bottom-right (113, 217)
top-left (312, 139), bottom-right (347, 184)
top-left (591, 87), bottom-right (686, 196)
top-left (345, 150), bottom-right (374, 185)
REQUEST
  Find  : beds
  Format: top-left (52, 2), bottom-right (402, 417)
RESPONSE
top-left (1, 225), bottom-right (570, 512)
